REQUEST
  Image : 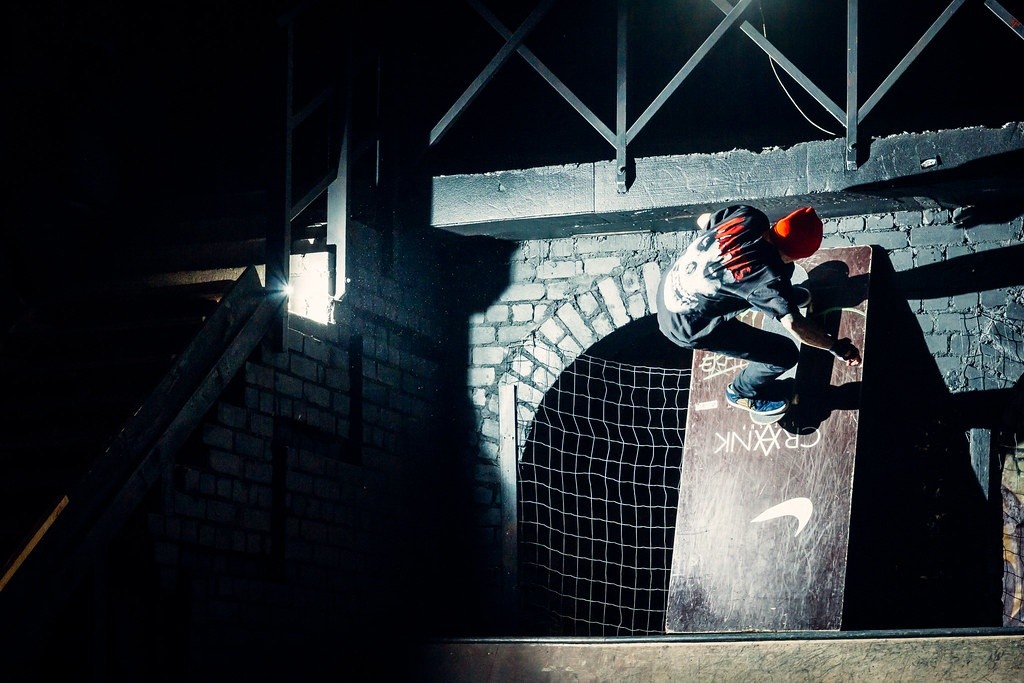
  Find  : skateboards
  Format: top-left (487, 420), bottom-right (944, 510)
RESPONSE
top-left (750, 262), bottom-right (810, 427)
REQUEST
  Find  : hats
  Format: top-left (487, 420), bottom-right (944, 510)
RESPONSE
top-left (772, 207), bottom-right (823, 260)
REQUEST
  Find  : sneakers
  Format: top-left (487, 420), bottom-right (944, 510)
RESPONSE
top-left (726, 381), bottom-right (789, 416)
top-left (789, 285), bottom-right (812, 309)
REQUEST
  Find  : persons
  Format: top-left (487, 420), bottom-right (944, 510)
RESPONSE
top-left (655, 204), bottom-right (861, 416)
